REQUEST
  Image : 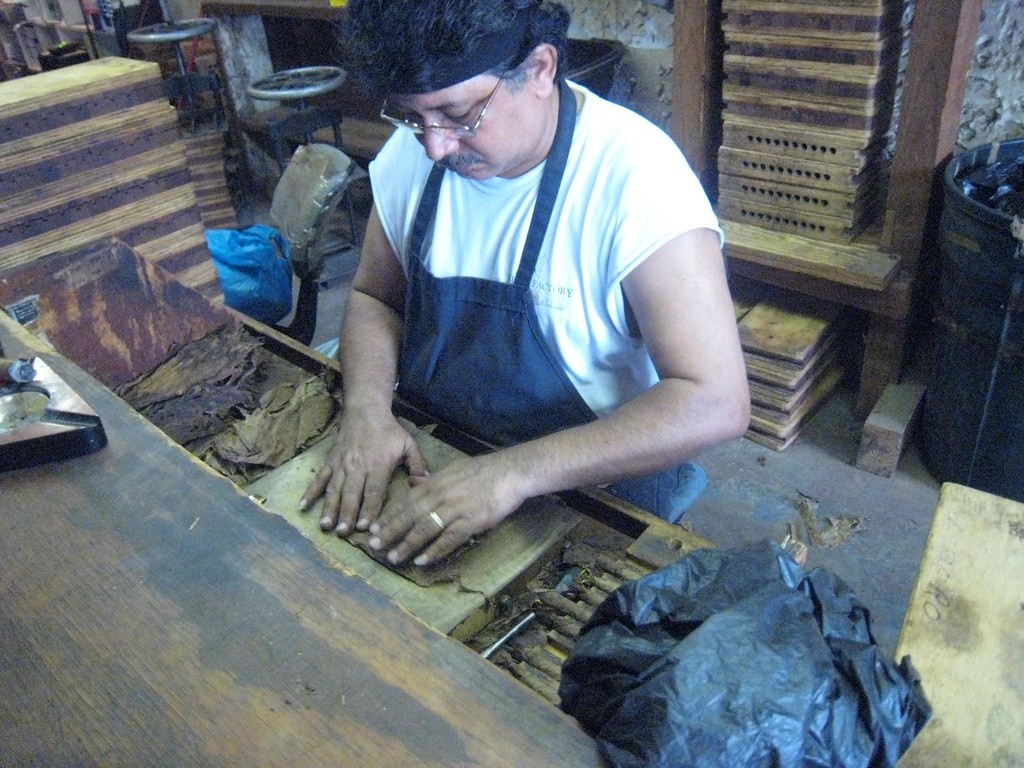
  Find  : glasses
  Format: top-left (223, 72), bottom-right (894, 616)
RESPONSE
top-left (380, 67), bottom-right (512, 142)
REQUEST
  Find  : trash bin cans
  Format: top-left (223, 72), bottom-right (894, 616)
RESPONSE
top-left (562, 34), bottom-right (628, 99)
top-left (916, 137), bottom-right (1024, 504)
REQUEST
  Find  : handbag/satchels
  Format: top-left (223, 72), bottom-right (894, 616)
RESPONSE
top-left (204, 224), bottom-right (292, 325)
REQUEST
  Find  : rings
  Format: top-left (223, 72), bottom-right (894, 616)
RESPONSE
top-left (428, 508), bottom-right (447, 530)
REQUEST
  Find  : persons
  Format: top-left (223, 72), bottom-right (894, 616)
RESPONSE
top-left (296, 1), bottom-right (752, 571)
